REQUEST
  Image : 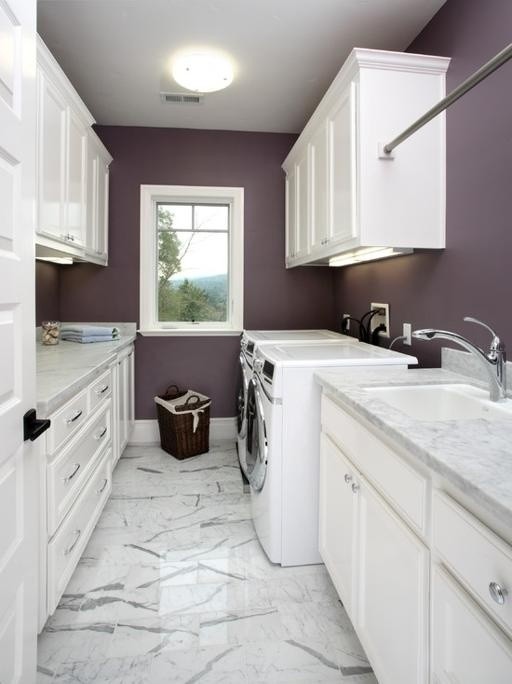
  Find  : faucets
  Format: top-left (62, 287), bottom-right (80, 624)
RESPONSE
top-left (411, 316), bottom-right (506, 402)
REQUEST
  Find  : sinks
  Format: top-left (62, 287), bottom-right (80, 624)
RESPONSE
top-left (358, 381), bottom-right (512, 423)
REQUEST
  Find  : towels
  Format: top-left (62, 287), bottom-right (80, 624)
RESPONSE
top-left (61, 328), bottom-right (120, 343)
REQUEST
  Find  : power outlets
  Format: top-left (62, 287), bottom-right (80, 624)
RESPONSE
top-left (344, 314), bottom-right (350, 330)
top-left (370, 303), bottom-right (390, 338)
top-left (403, 323), bottom-right (412, 346)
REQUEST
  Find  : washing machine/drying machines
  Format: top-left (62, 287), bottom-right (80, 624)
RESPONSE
top-left (236, 328), bottom-right (360, 494)
top-left (245, 341), bottom-right (419, 568)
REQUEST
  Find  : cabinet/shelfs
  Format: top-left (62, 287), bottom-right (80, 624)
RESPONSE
top-left (114, 342), bottom-right (136, 461)
top-left (317, 393), bottom-right (428, 684)
top-left (45, 365), bottom-right (114, 621)
top-left (86, 127), bottom-right (114, 268)
top-left (281, 137), bottom-right (312, 271)
top-left (37, 64), bottom-right (87, 256)
top-left (428, 483), bottom-right (512, 683)
top-left (315, 46), bottom-right (453, 249)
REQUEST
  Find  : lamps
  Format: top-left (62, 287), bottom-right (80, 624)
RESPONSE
top-left (170, 50), bottom-right (237, 93)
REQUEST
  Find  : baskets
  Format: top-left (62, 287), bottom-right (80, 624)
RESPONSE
top-left (153, 385), bottom-right (212, 459)
